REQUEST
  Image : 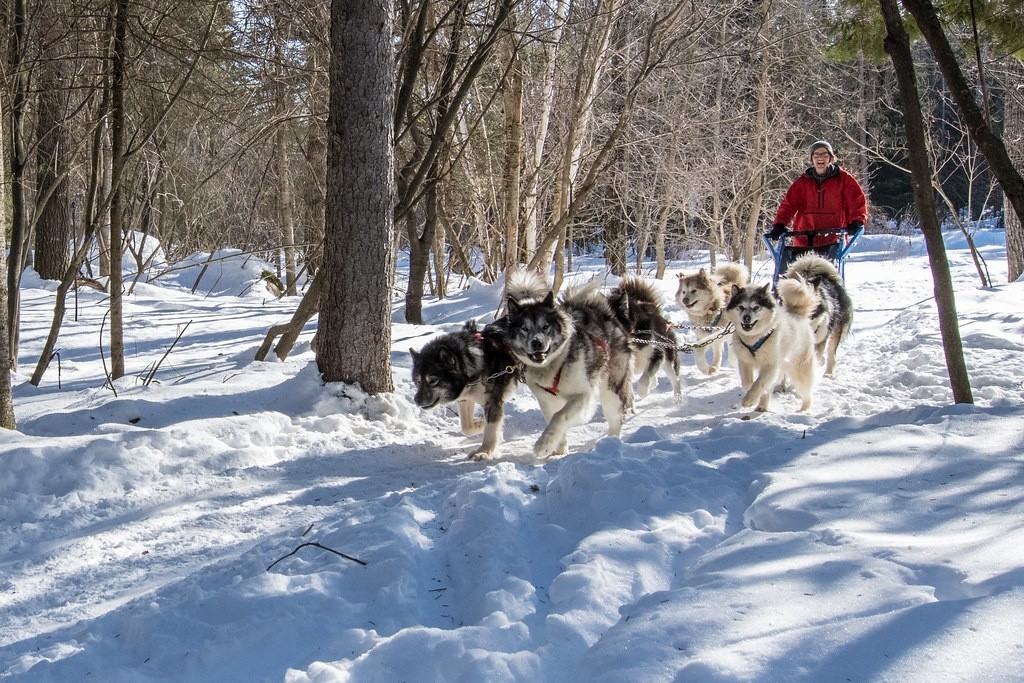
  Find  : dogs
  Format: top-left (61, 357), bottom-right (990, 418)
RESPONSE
top-left (727, 279), bottom-right (821, 412)
top-left (408, 266), bottom-right (551, 461)
top-left (783, 249), bottom-right (854, 379)
top-left (675, 262), bottom-right (750, 374)
top-left (504, 291), bottom-right (636, 460)
top-left (597, 276), bottom-right (681, 398)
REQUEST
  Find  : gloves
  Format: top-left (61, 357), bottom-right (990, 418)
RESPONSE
top-left (770, 223), bottom-right (788, 241)
top-left (845, 220), bottom-right (860, 235)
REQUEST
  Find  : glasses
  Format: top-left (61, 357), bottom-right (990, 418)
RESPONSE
top-left (813, 152), bottom-right (829, 157)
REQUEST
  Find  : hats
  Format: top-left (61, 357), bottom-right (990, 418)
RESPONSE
top-left (809, 141), bottom-right (835, 160)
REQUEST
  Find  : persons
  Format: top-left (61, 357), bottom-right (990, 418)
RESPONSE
top-left (770, 141), bottom-right (867, 265)
